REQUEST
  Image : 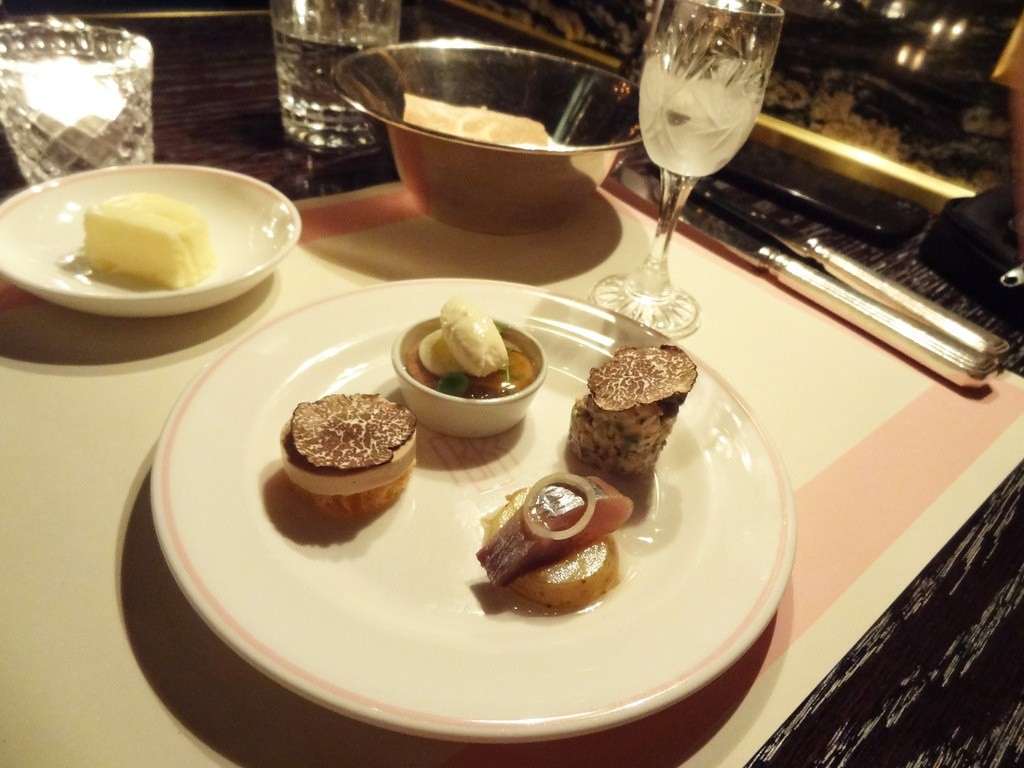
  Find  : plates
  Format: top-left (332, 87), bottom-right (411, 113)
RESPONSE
top-left (149, 278), bottom-right (799, 744)
top-left (0, 164), bottom-right (302, 318)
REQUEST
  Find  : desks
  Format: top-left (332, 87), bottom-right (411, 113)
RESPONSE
top-left (0, 181), bottom-right (1024, 768)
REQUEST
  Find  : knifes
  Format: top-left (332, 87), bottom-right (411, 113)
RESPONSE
top-left (694, 184), bottom-right (1010, 363)
top-left (648, 177), bottom-right (998, 389)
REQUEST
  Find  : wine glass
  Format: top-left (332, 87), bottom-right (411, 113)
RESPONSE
top-left (589, 0), bottom-right (785, 341)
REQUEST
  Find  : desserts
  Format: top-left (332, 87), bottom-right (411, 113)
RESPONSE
top-left (417, 295), bottom-right (508, 376)
top-left (84, 192), bottom-right (216, 288)
top-left (283, 395), bottom-right (419, 515)
top-left (571, 343), bottom-right (695, 477)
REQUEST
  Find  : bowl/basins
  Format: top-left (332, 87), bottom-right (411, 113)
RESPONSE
top-left (330, 40), bottom-right (643, 233)
top-left (391, 317), bottom-right (548, 438)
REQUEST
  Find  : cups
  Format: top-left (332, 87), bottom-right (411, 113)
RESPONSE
top-left (0, 21), bottom-right (154, 185)
top-left (269, 0), bottom-right (401, 155)
